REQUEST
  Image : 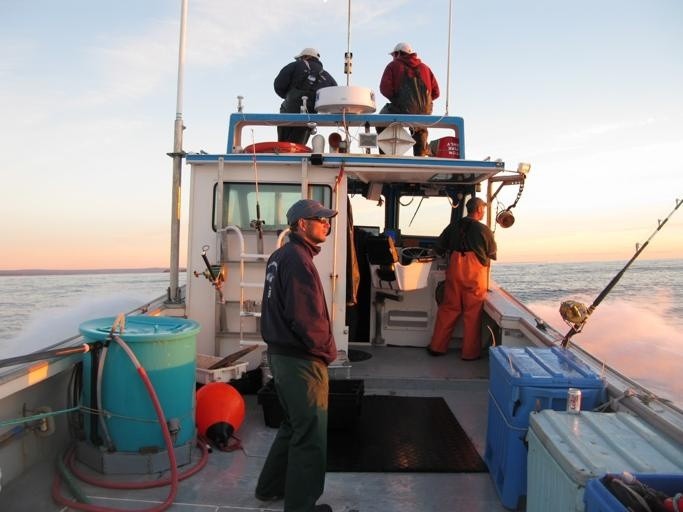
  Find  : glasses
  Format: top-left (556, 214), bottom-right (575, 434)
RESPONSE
top-left (304, 216), bottom-right (327, 224)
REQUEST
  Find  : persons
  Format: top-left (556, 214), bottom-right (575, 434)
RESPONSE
top-left (375, 42), bottom-right (441, 158)
top-left (426, 198), bottom-right (499, 361)
top-left (252, 199), bottom-right (339, 511)
top-left (272, 46), bottom-right (338, 148)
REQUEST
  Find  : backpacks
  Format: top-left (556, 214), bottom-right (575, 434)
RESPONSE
top-left (396, 58), bottom-right (427, 114)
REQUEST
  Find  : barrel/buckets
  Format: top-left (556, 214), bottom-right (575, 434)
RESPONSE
top-left (79, 315), bottom-right (201, 451)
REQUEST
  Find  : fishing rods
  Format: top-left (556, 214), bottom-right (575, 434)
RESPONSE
top-left (561, 198), bottom-right (683, 347)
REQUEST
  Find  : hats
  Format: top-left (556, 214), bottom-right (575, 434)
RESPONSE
top-left (388, 41), bottom-right (416, 55)
top-left (466, 197), bottom-right (488, 212)
top-left (293, 47), bottom-right (321, 61)
top-left (285, 199), bottom-right (338, 225)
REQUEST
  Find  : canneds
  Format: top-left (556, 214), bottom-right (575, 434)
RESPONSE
top-left (567, 387), bottom-right (581, 415)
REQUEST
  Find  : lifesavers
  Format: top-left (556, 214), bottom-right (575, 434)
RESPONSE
top-left (244, 142), bottom-right (311, 153)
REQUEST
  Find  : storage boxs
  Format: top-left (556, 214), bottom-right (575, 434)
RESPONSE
top-left (583, 473), bottom-right (683, 511)
top-left (526, 409), bottom-right (683, 511)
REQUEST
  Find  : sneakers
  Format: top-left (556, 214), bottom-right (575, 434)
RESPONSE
top-left (432, 352), bottom-right (445, 356)
top-left (283, 503), bottom-right (333, 512)
top-left (463, 356), bottom-right (480, 360)
